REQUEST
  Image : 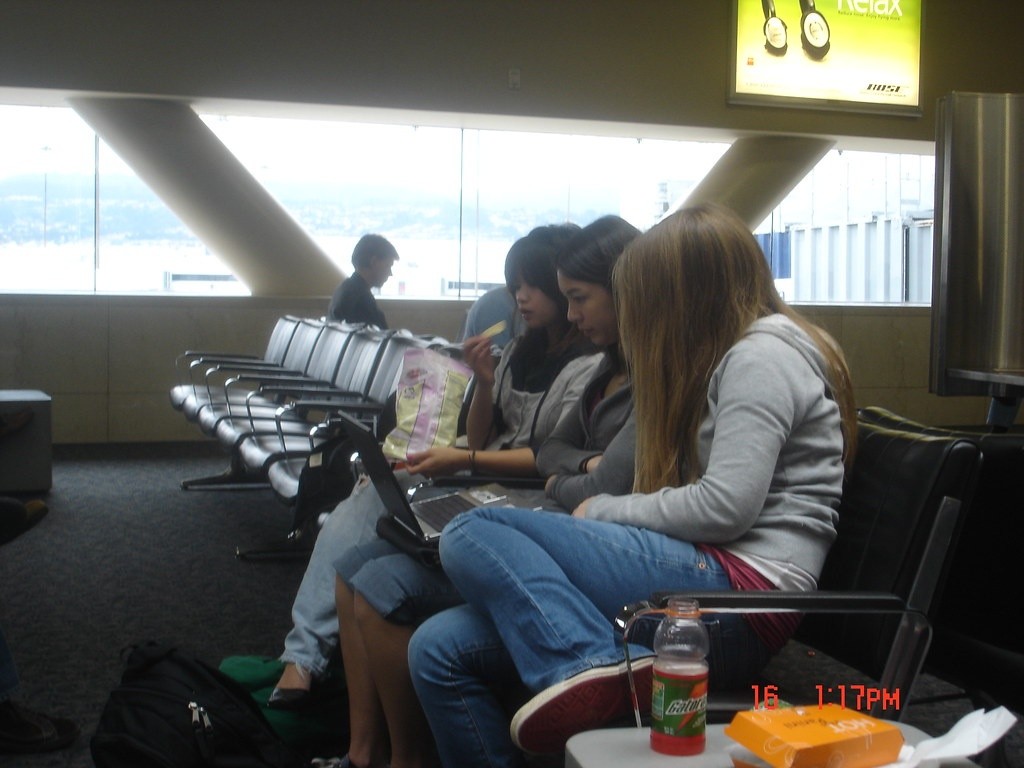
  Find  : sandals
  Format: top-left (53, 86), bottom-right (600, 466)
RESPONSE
top-left (0, 700), bottom-right (83, 753)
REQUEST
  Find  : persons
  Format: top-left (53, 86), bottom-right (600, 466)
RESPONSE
top-left (267, 200), bottom-right (860, 768)
top-left (328, 235), bottom-right (399, 328)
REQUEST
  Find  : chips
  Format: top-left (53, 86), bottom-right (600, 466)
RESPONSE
top-left (483, 320), bottom-right (507, 336)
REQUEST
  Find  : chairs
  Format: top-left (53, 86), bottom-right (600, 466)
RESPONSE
top-left (237, 330), bottom-right (447, 468)
top-left (197, 322), bottom-right (359, 435)
top-left (267, 339), bottom-right (474, 502)
top-left (180, 318), bottom-right (326, 422)
top-left (216, 326), bottom-right (393, 448)
top-left (613, 420), bottom-right (985, 729)
top-left (168, 314), bottom-right (303, 413)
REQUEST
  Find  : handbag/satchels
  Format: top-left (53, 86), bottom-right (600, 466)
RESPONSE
top-left (90, 638), bottom-right (306, 768)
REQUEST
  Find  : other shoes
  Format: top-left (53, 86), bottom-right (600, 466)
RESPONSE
top-left (265, 685), bottom-right (309, 710)
top-left (509, 656), bottom-right (661, 758)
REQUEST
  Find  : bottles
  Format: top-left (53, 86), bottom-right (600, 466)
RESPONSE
top-left (649, 596), bottom-right (708, 755)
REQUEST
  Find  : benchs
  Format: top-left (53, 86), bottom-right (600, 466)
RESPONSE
top-left (861, 406), bottom-right (1024, 719)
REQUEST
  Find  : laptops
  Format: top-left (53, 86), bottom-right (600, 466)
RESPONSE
top-left (339, 409), bottom-right (542, 545)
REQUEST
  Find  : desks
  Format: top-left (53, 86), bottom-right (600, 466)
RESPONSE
top-left (564, 721), bottom-right (981, 768)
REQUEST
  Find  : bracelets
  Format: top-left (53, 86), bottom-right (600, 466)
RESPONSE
top-left (471, 449), bottom-right (477, 475)
top-left (581, 457), bottom-right (588, 473)
